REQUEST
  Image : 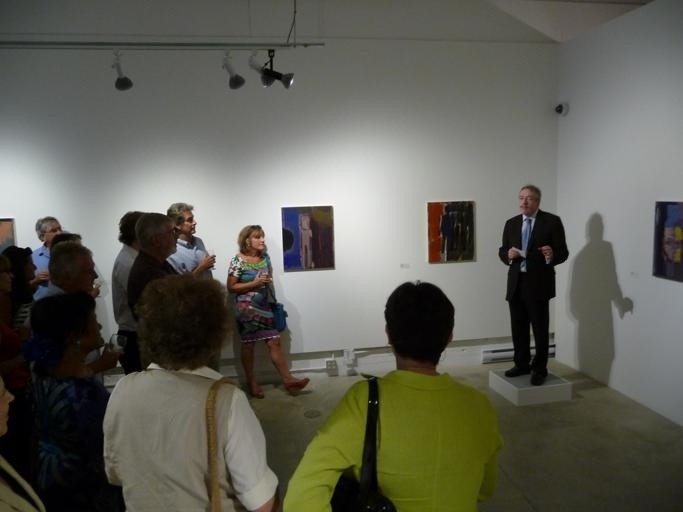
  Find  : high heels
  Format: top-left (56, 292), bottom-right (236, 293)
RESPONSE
top-left (284, 378), bottom-right (310, 395)
top-left (250, 387), bottom-right (264, 398)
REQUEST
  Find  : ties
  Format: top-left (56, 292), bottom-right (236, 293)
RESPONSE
top-left (520, 220), bottom-right (531, 269)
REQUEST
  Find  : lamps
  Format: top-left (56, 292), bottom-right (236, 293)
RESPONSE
top-left (248, 46), bottom-right (297, 92)
top-left (222, 50), bottom-right (245, 90)
top-left (111, 54), bottom-right (133, 90)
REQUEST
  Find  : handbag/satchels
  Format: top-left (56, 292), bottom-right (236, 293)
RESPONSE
top-left (273, 303), bottom-right (287, 332)
top-left (330, 468), bottom-right (397, 512)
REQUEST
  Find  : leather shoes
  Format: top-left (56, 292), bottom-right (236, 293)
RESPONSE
top-left (505, 365), bottom-right (530, 378)
top-left (530, 368), bottom-right (548, 386)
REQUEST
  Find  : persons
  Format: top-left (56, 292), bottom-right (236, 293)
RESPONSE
top-left (112, 213), bottom-right (146, 373)
top-left (0, 217), bottom-right (124, 512)
top-left (227, 224), bottom-right (309, 399)
top-left (127, 214), bottom-right (182, 323)
top-left (101, 274), bottom-right (280, 511)
top-left (500, 185), bottom-right (569, 386)
top-left (281, 283), bottom-right (504, 512)
top-left (166, 203), bottom-right (216, 281)
